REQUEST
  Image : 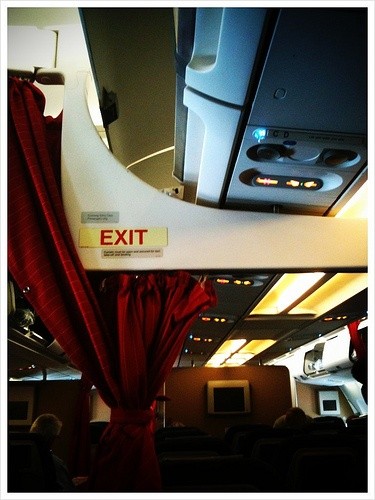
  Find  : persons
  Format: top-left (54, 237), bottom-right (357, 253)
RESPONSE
top-left (29, 413), bottom-right (67, 464)
top-left (273, 407), bottom-right (311, 440)
